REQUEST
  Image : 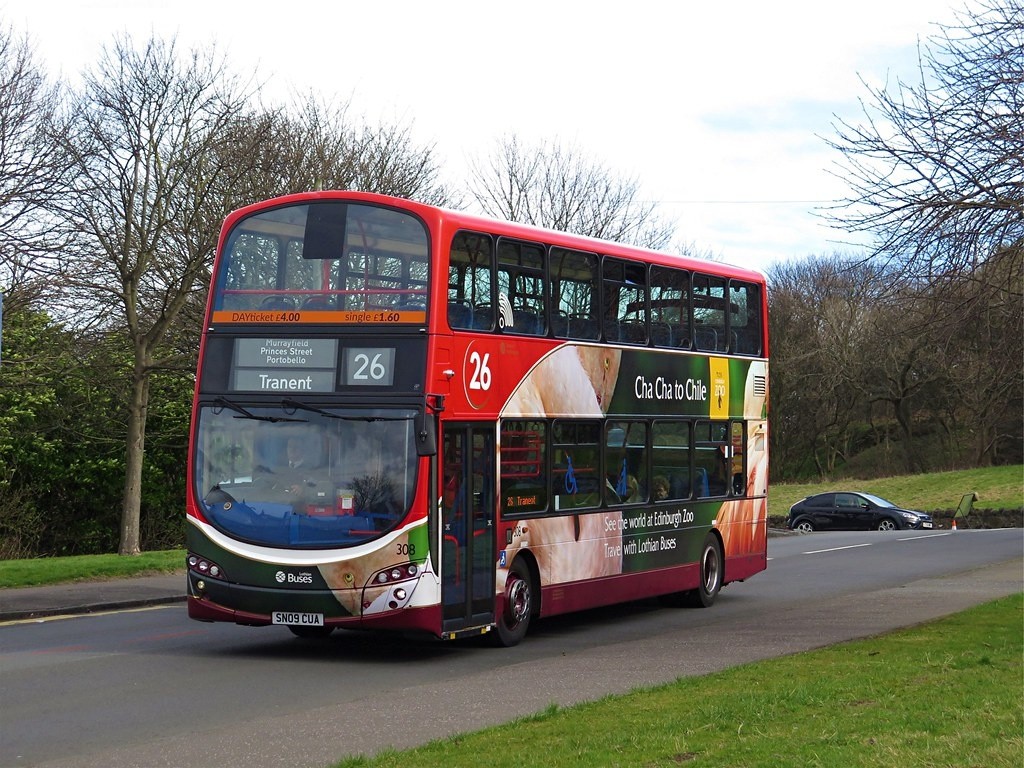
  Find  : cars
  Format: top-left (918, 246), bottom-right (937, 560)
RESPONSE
top-left (785, 491), bottom-right (935, 531)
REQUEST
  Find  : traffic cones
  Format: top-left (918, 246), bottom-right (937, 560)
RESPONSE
top-left (951, 520), bottom-right (957, 531)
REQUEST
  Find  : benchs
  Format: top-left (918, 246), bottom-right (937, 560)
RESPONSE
top-left (651, 465), bottom-right (709, 500)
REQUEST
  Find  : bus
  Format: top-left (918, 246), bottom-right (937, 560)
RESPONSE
top-left (185, 190), bottom-right (771, 648)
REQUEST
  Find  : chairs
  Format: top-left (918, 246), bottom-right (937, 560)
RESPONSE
top-left (261, 295), bottom-right (755, 353)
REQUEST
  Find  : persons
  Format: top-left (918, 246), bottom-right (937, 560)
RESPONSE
top-left (268, 437), bottom-right (315, 494)
top-left (482, 436), bottom-right (671, 508)
